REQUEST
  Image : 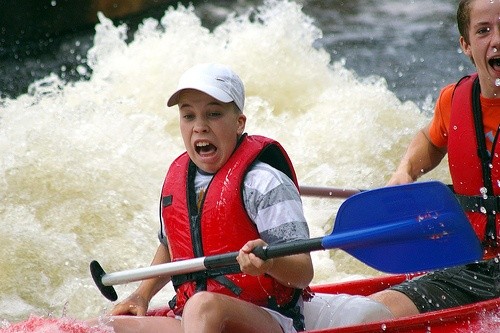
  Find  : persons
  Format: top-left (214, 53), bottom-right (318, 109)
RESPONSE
top-left (79, 62), bottom-right (314, 333)
top-left (363, 0), bottom-right (500, 319)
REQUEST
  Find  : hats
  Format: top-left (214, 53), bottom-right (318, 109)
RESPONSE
top-left (167, 65), bottom-right (246, 113)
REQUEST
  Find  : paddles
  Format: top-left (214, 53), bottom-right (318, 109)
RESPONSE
top-left (90, 181), bottom-right (484, 302)
top-left (299, 186), bottom-right (369, 198)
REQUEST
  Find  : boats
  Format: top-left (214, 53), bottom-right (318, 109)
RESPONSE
top-left (2, 272), bottom-right (500, 333)
